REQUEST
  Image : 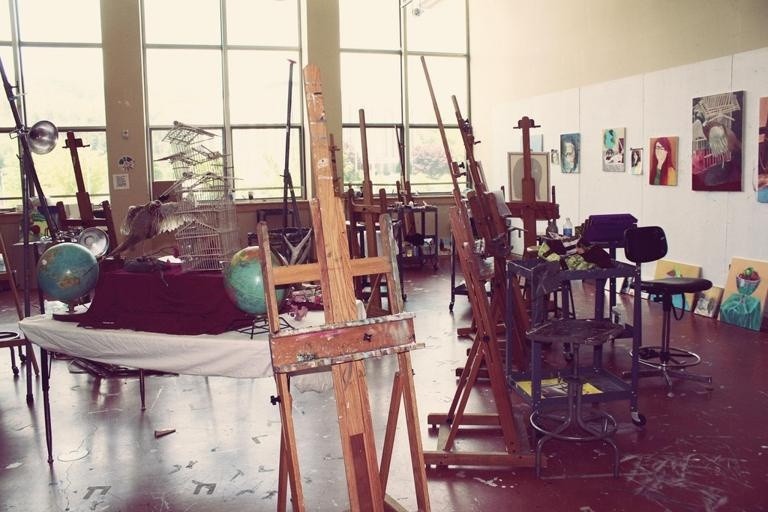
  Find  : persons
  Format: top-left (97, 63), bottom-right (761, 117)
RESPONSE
top-left (650, 136), bottom-right (677, 184)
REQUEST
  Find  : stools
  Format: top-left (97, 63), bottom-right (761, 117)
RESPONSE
top-left (525, 317), bottom-right (623, 479)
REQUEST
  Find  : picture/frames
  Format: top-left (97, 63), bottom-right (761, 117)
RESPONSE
top-left (508, 151), bottom-right (549, 202)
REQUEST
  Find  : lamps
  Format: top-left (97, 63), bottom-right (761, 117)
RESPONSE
top-left (10, 120), bottom-right (59, 154)
top-left (58, 227), bottom-right (109, 259)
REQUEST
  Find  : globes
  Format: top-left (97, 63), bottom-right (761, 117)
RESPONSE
top-left (223, 246), bottom-right (297, 339)
top-left (37, 243), bottom-right (100, 321)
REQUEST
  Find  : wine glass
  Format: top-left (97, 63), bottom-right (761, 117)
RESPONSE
top-left (734, 272), bottom-right (761, 313)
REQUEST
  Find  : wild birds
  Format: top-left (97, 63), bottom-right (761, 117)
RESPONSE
top-left (107, 199), bottom-right (208, 258)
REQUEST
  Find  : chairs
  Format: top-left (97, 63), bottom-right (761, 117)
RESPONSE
top-left (622, 226), bottom-right (714, 398)
top-left (583, 213), bottom-right (637, 318)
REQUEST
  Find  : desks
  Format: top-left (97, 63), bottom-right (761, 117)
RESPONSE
top-left (22, 299), bottom-right (370, 464)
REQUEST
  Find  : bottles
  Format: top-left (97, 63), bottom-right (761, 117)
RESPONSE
top-left (562, 217), bottom-right (573, 237)
